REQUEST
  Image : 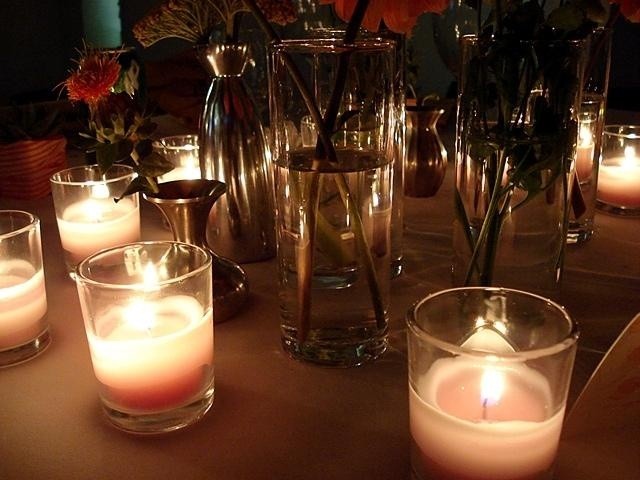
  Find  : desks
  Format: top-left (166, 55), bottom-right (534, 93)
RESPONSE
top-left (4, 101), bottom-right (637, 480)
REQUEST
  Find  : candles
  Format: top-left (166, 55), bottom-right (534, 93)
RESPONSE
top-left (75, 240), bottom-right (214, 433)
top-left (599, 120), bottom-right (639, 211)
top-left (406, 279), bottom-right (577, 480)
top-left (50, 167), bottom-right (140, 280)
top-left (4, 209), bottom-right (51, 368)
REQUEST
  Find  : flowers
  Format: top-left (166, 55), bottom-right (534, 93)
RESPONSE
top-left (131, 2), bottom-right (606, 341)
top-left (69, 45), bottom-right (196, 197)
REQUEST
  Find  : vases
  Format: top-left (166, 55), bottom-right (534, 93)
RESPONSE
top-left (4, 129), bottom-right (72, 205)
top-left (142, 36), bottom-right (612, 361)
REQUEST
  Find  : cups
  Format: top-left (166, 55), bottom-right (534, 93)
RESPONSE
top-left (154, 133), bottom-right (199, 228)
top-left (50, 164), bottom-right (141, 282)
top-left (264, 114), bottom-right (317, 157)
top-left (75, 240), bottom-right (216, 437)
top-left (576, 113), bottom-right (640, 215)
top-left (406, 288), bottom-right (580, 480)
top-left (0, 209), bottom-right (52, 369)
